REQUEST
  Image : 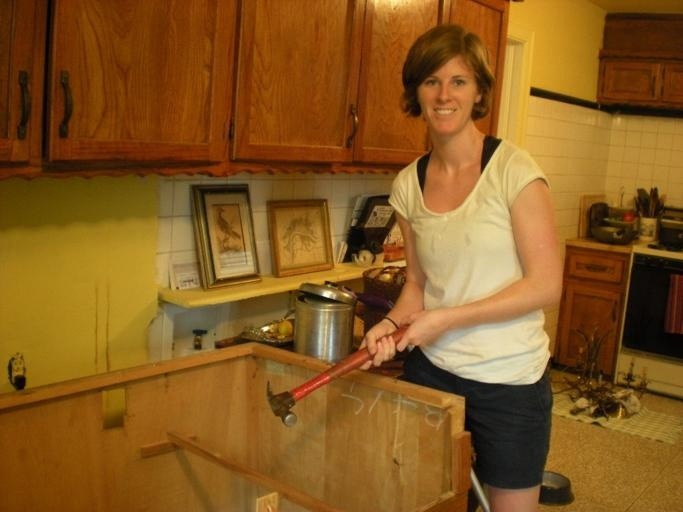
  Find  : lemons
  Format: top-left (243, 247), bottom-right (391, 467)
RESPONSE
top-left (278, 320), bottom-right (293, 337)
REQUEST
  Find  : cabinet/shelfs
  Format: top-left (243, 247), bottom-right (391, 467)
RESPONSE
top-left (0, 0), bottom-right (510, 174)
top-left (550, 244), bottom-right (631, 380)
top-left (598, 12), bottom-right (682, 111)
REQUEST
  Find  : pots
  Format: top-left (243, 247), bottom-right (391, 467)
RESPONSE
top-left (592, 226), bottom-right (636, 245)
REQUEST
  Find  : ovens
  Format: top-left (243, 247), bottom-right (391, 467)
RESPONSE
top-left (621, 253), bottom-right (683, 361)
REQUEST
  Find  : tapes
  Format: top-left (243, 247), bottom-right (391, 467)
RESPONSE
top-left (358, 249), bottom-right (372, 263)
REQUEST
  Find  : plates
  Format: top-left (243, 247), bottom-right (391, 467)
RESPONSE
top-left (588, 204), bottom-right (610, 237)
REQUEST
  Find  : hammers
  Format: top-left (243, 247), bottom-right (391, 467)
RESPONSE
top-left (267, 322), bottom-right (414, 426)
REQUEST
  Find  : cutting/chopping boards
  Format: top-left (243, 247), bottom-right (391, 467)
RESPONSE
top-left (580, 191), bottom-right (609, 240)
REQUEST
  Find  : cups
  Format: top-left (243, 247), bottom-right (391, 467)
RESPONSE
top-left (638, 217), bottom-right (657, 241)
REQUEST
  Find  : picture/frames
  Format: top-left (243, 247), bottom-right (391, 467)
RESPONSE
top-left (266, 199), bottom-right (334, 278)
top-left (190, 183), bottom-right (262, 291)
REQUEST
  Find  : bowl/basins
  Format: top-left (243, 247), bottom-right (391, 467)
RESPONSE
top-left (362, 267), bottom-right (405, 299)
top-left (539, 469), bottom-right (575, 506)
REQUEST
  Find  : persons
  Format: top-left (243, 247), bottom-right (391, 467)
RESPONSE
top-left (359, 23), bottom-right (564, 512)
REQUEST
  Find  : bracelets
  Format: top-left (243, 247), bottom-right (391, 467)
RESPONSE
top-left (384, 317), bottom-right (399, 330)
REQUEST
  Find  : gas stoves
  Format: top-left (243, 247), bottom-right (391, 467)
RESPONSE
top-left (633, 237), bottom-right (683, 260)
